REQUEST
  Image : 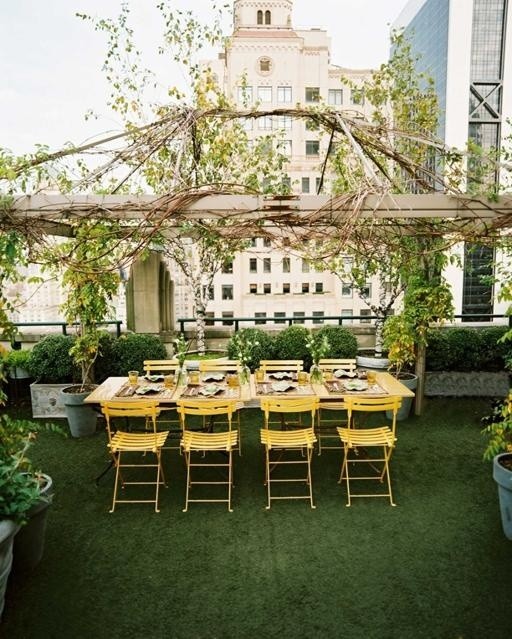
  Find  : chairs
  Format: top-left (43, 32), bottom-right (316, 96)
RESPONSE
top-left (259, 397), bottom-right (319, 510)
top-left (336, 395), bottom-right (399, 508)
top-left (142, 360), bottom-right (186, 457)
top-left (258, 359), bottom-right (306, 458)
top-left (309, 359), bottom-right (361, 457)
top-left (176, 400), bottom-right (239, 511)
top-left (197, 361), bottom-right (243, 457)
top-left (101, 398), bottom-right (169, 512)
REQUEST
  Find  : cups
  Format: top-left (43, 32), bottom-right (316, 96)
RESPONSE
top-left (186, 371), bottom-right (201, 385)
top-left (358, 367), bottom-right (377, 385)
top-left (225, 373), bottom-right (239, 387)
top-left (164, 375), bottom-right (174, 391)
top-left (128, 370), bottom-right (139, 385)
top-left (255, 368), bottom-right (267, 384)
top-left (324, 370), bottom-right (334, 380)
top-left (297, 371), bottom-right (308, 385)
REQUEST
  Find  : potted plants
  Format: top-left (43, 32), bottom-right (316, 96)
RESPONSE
top-left (39, 218), bottom-right (129, 439)
top-left (288, 21), bottom-right (465, 371)
top-left (26, 335), bottom-right (81, 419)
top-left (75, 1), bottom-right (257, 361)
top-left (478, 284), bottom-right (512, 539)
top-left (412, 326), bottom-right (510, 397)
top-left (379, 273), bottom-right (455, 421)
top-left (0, 415), bottom-right (53, 615)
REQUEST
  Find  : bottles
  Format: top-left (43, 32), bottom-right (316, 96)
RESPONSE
top-left (174, 366), bottom-right (187, 387)
top-left (310, 364), bottom-right (323, 385)
top-left (239, 364), bottom-right (251, 385)
